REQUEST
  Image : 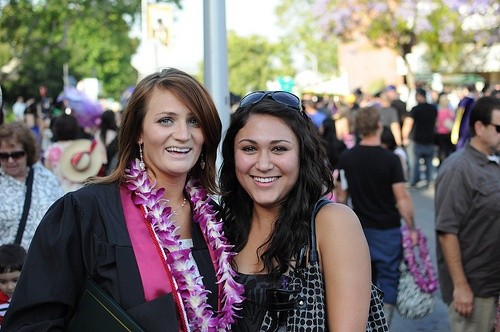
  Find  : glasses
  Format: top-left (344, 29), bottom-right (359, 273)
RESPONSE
top-left (239, 91), bottom-right (303, 113)
top-left (0, 150), bottom-right (28, 160)
top-left (490, 122), bottom-right (500, 132)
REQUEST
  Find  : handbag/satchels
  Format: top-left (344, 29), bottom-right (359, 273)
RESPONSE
top-left (260, 198), bottom-right (389, 332)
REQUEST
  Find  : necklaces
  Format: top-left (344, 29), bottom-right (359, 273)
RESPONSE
top-left (173, 196), bottom-right (187, 213)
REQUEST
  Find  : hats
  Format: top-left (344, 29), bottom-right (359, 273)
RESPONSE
top-left (61, 139), bottom-right (106, 184)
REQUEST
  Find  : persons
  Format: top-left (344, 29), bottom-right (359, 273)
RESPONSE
top-left (434, 95), bottom-right (500, 332)
top-left (0, 120), bottom-right (65, 252)
top-left (0, 67), bottom-right (245, 332)
top-left (0, 81), bottom-right (500, 203)
top-left (334, 107), bottom-right (414, 332)
top-left (218, 91), bottom-right (391, 332)
top-left (0, 244), bottom-right (27, 331)
